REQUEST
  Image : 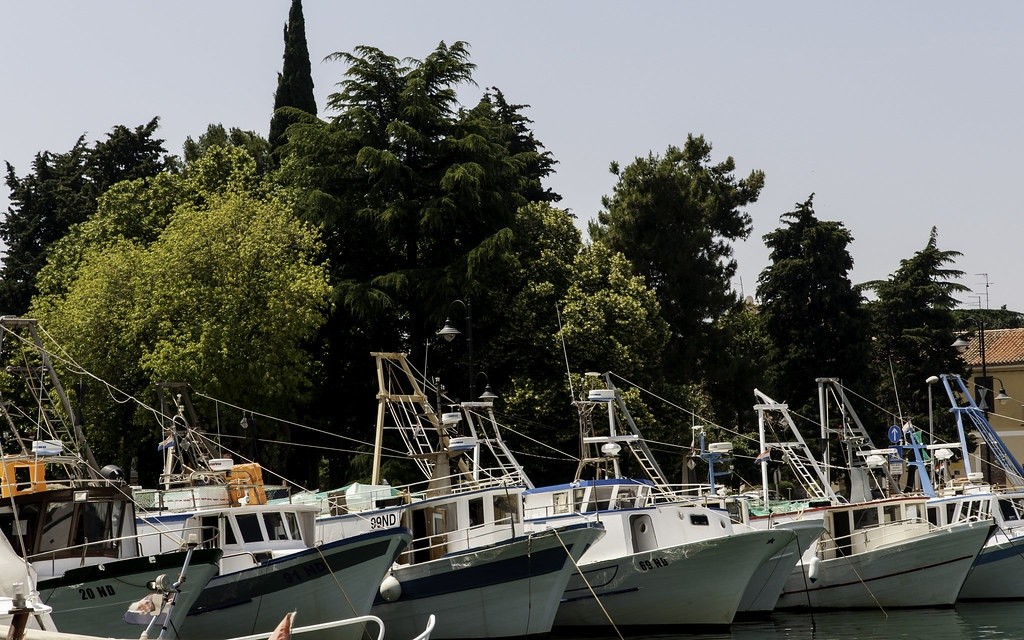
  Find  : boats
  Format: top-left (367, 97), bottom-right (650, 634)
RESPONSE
top-left (0, 314), bottom-right (1024, 640)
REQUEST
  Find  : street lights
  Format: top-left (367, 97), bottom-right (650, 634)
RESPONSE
top-left (437, 300), bottom-right (498, 419)
top-left (951, 316), bottom-right (1010, 483)
